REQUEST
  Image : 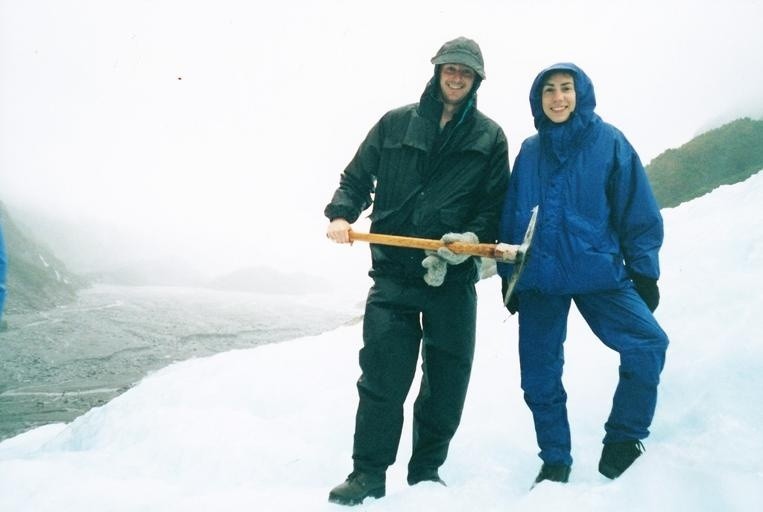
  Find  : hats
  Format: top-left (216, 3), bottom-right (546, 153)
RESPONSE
top-left (430, 35), bottom-right (490, 81)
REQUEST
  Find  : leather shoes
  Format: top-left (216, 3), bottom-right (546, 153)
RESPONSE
top-left (328, 462), bottom-right (387, 506)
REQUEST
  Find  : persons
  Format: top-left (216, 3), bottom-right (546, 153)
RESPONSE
top-left (324, 36), bottom-right (510, 505)
top-left (496, 61), bottom-right (663, 490)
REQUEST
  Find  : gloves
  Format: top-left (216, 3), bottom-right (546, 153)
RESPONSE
top-left (498, 278), bottom-right (521, 315)
top-left (419, 247), bottom-right (448, 289)
top-left (635, 276), bottom-right (661, 316)
top-left (438, 231), bottom-right (479, 265)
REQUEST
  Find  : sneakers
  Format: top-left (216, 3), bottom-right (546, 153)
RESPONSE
top-left (597, 439), bottom-right (643, 478)
top-left (529, 457), bottom-right (572, 493)
top-left (405, 467), bottom-right (446, 491)
